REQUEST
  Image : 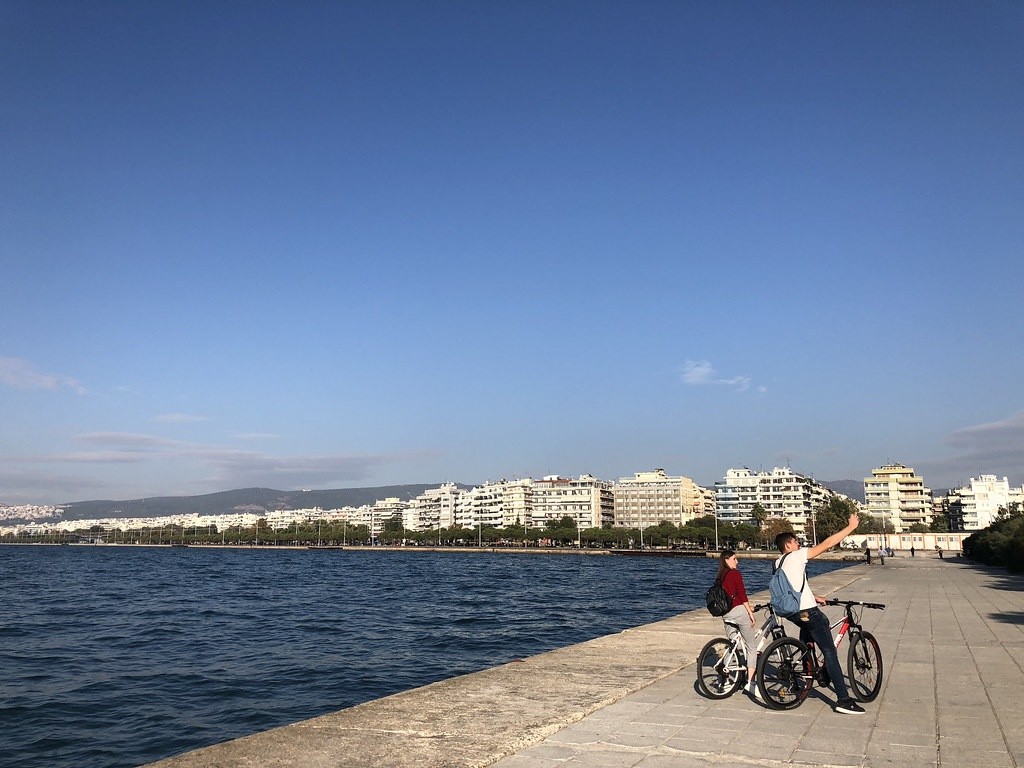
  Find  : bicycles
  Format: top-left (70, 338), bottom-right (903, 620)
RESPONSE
top-left (697, 602), bottom-right (799, 699)
top-left (756, 597), bottom-right (885, 711)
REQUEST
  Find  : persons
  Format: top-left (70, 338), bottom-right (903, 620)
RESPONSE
top-left (885, 547), bottom-right (895, 557)
top-left (911, 546), bottom-right (914, 557)
top-left (718, 550), bottom-right (765, 699)
top-left (938, 548), bottom-right (942, 558)
top-left (878, 546), bottom-right (887, 565)
top-left (863, 545), bottom-right (871, 565)
top-left (773, 513), bottom-right (866, 714)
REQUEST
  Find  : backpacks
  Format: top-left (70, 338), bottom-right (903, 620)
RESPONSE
top-left (769, 553), bottom-right (806, 617)
top-left (706, 570), bottom-right (738, 617)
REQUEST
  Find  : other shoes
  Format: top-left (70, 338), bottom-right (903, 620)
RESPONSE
top-left (744, 684), bottom-right (763, 699)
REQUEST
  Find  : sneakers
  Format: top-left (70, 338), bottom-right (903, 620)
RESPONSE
top-left (835, 697), bottom-right (866, 714)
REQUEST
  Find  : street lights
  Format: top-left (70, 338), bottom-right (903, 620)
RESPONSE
top-left (711, 495), bottom-right (718, 551)
top-left (640, 520), bottom-right (643, 544)
top-left (882, 502), bottom-right (887, 548)
